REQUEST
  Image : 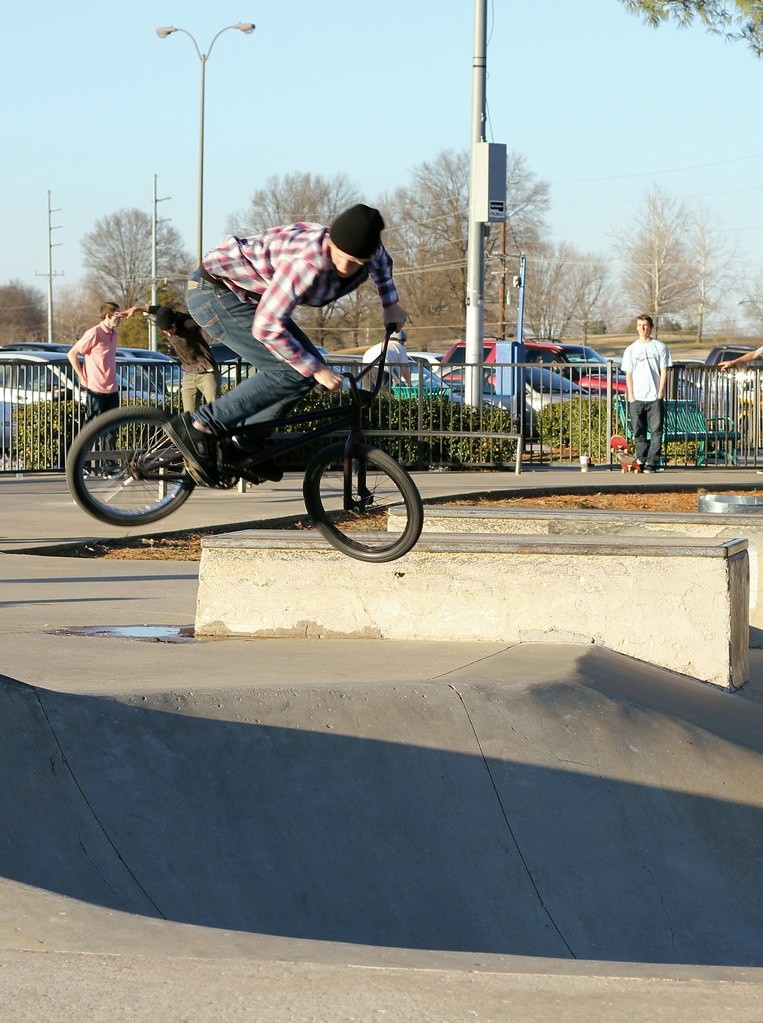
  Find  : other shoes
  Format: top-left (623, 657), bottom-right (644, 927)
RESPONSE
top-left (636, 462), bottom-right (644, 472)
top-left (103, 470), bottom-right (122, 480)
top-left (643, 461), bottom-right (656, 474)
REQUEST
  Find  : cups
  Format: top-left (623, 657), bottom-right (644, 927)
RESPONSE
top-left (580, 456), bottom-right (589, 472)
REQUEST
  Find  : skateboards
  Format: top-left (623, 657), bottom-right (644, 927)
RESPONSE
top-left (610, 435), bottom-right (641, 473)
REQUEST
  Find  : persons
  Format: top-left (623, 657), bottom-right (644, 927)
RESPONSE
top-left (162, 205), bottom-right (408, 487)
top-left (528, 351), bottom-right (567, 376)
top-left (116, 305), bottom-right (221, 413)
top-left (718, 345), bottom-right (763, 370)
top-left (361, 330), bottom-right (411, 393)
top-left (66, 302), bottom-right (122, 479)
top-left (621, 313), bottom-right (673, 473)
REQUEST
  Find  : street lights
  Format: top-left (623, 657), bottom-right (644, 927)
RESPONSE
top-left (149, 276), bottom-right (175, 351)
top-left (155, 24), bottom-right (258, 268)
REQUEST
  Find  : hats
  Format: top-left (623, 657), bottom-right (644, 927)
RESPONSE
top-left (155, 307), bottom-right (176, 330)
top-left (330, 204), bottom-right (385, 260)
top-left (387, 329), bottom-right (408, 340)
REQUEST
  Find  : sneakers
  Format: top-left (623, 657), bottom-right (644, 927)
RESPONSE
top-left (162, 412), bottom-right (219, 489)
top-left (234, 433), bottom-right (283, 482)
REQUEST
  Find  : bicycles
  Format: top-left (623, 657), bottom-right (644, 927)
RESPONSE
top-left (63, 323), bottom-right (425, 563)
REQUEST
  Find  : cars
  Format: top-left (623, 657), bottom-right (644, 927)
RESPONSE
top-left (0, 341), bottom-right (600, 456)
top-left (672, 360), bottom-right (706, 399)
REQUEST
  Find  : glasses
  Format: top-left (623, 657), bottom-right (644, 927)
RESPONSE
top-left (539, 358), bottom-right (543, 361)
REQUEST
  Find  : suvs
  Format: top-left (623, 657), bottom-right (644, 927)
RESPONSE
top-left (438, 337), bottom-right (698, 400)
top-left (699, 345), bottom-right (763, 408)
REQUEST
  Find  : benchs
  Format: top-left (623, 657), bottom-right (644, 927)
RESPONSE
top-left (612, 394), bottom-right (741, 471)
